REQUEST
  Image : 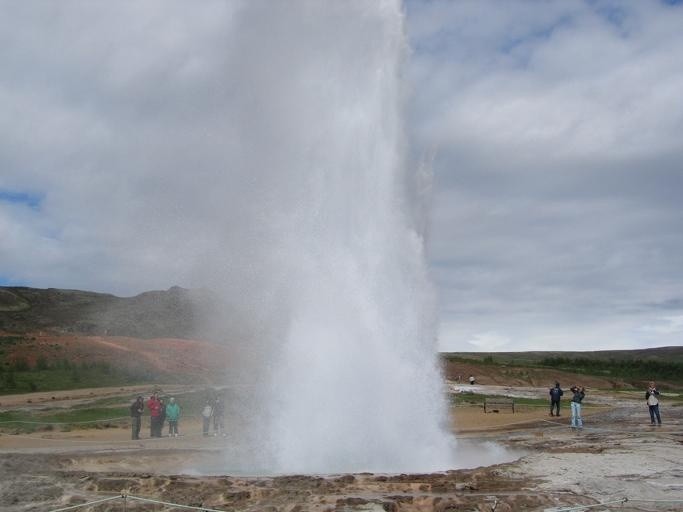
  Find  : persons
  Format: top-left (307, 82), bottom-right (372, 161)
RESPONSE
top-left (549, 383), bottom-right (563, 417)
top-left (468, 375), bottom-right (475, 384)
top-left (569, 384), bottom-right (585, 428)
top-left (645, 381), bottom-right (662, 424)
top-left (130, 392), bottom-right (228, 439)
